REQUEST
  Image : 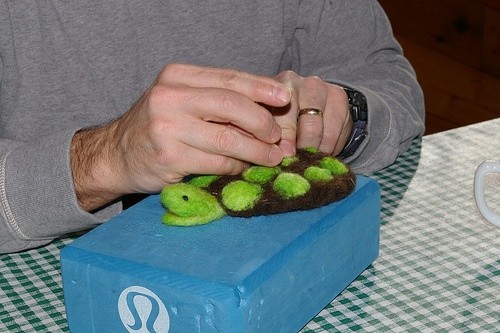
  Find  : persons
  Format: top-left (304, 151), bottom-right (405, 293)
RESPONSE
top-left (0, 0), bottom-right (425, 254)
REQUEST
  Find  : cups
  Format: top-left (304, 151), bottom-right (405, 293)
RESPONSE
top-left (474, 159), bottom-right (500, 228)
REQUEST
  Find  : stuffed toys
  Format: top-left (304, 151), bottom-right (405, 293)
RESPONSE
top-left (160, 147), bottom-right (356, 226)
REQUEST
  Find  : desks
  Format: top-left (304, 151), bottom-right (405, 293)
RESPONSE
top-left (0, 118), bottom-right (500, 333)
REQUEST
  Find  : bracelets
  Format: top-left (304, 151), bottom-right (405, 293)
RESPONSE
top-left (328, 81), bottom-right (368, 158)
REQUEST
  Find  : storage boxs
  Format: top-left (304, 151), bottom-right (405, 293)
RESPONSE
top-left (59, 174), bottom-right (381, 333)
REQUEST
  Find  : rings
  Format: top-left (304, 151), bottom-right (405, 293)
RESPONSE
top-left (298, 107), bottom-right (323, 117)
